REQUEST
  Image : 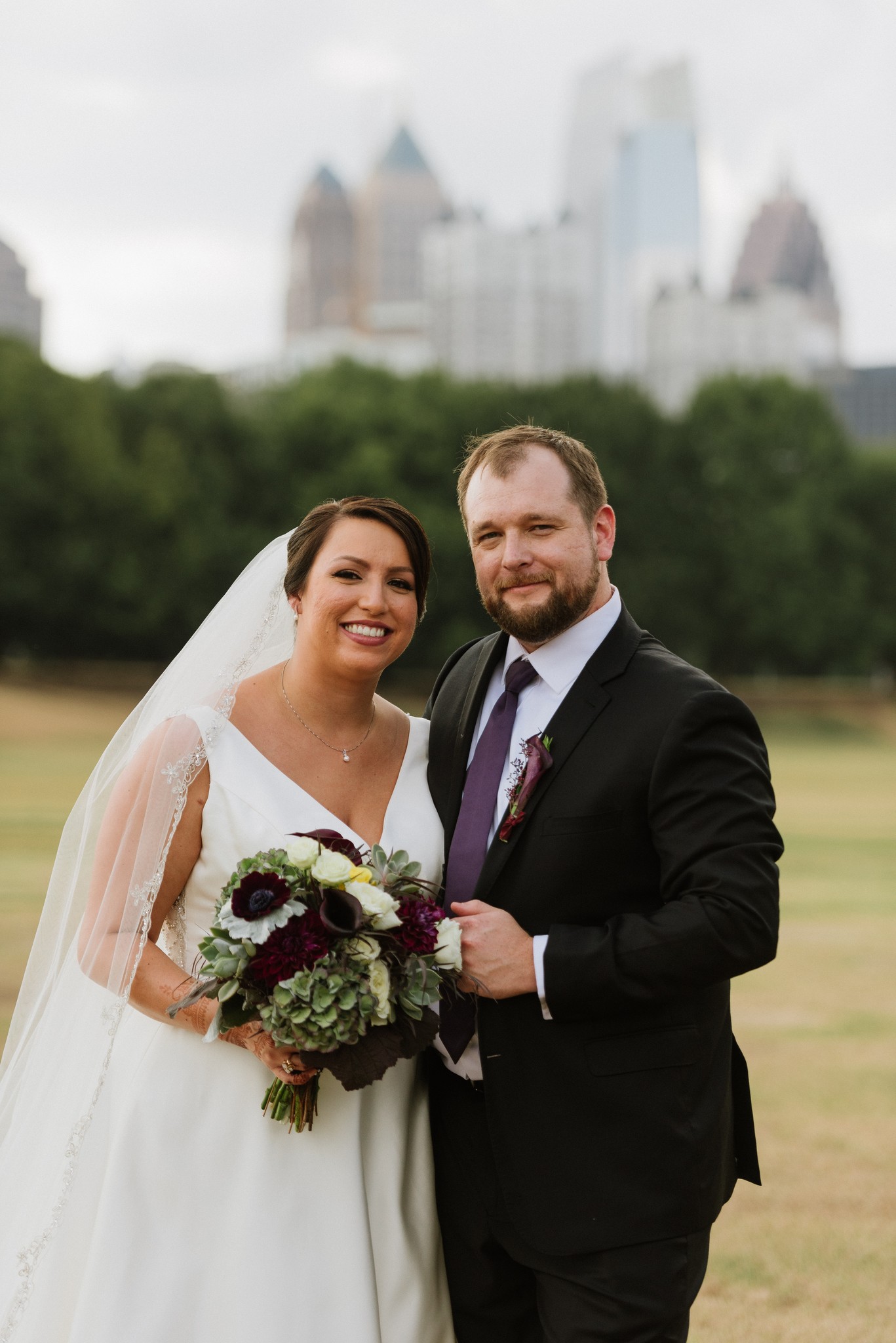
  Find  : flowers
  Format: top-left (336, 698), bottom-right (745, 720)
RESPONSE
top-left (495, 730), bottom-right (557, 841)
top-left (156, 828), bottom-right (464, 1134)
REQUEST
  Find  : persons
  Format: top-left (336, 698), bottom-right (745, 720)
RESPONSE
top-left (0, 498), bottom-right (456, 1343)
top-left (419, 426), bottom-right (786, 1343)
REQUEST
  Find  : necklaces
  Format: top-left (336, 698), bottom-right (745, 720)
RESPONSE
top-left (281, 661), bottom-right (375, 761)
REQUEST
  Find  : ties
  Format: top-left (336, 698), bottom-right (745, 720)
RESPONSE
top-left (439, 658), bottom-right (537, 1065)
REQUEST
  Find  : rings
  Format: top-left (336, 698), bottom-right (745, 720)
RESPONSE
top-left (282, 1057), bottom-right (294, 1074)
